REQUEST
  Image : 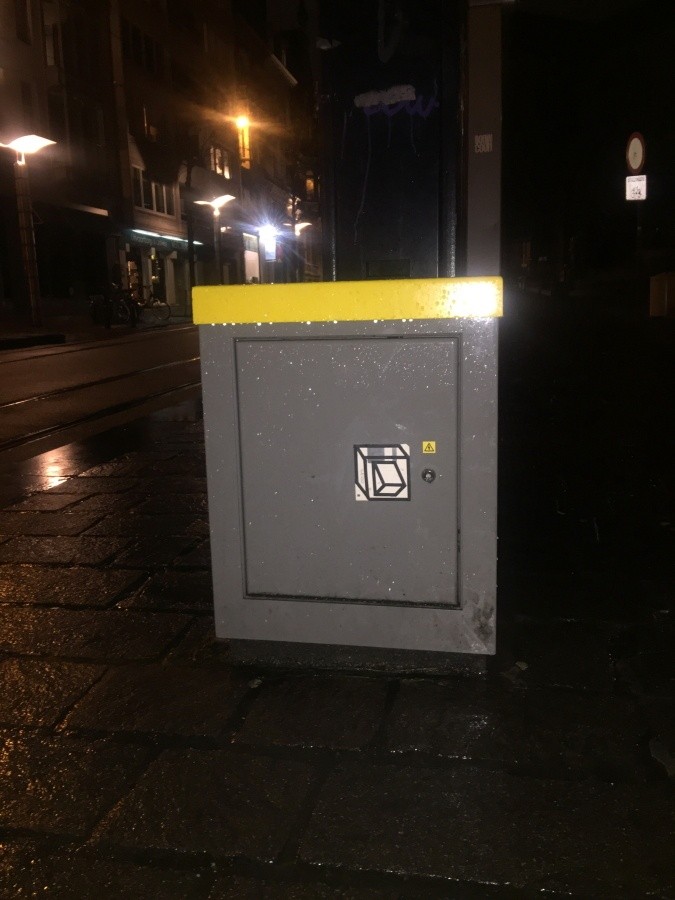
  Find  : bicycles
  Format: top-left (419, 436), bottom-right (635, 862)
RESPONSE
top-left (84, 283), bottom-right (173, 327)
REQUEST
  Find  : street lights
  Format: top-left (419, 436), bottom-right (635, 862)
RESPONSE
top-left (0, 130), bottom-right (58, 330)
top-left (286, 221), bottom-right (314, 282)
top-left (192, 193), bottom-right (236, 284)
